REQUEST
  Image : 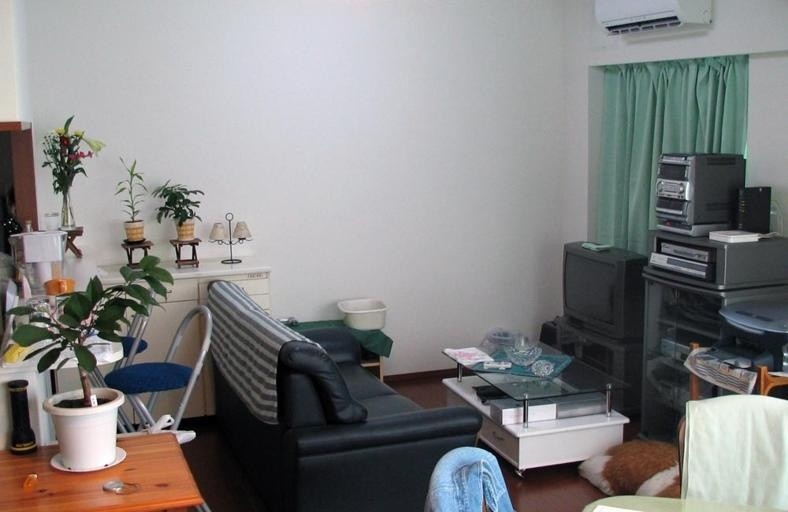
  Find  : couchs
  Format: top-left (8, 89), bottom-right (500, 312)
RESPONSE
top-left (204, 281), bottom-right (480, 512)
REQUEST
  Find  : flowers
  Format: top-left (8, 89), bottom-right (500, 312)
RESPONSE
top-left (39, 114), bottom-right (107, 225)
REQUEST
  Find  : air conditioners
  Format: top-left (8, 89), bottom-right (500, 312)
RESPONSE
top-left (594, 1), bottom-right (712, 44)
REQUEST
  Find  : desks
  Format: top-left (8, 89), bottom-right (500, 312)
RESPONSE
top-left (1, 324), bottom-right (123, 447)
top-left (639, 273), bottom-right (784, 435)
top-left (0, 432), bottom-right (205, 511)
top-left (94, 256), bottom-right (276, 428)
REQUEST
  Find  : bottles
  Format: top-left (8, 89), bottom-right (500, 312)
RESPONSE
top-left (44, 212), bottom-right (60, 231)
top-left (23, 219), bottom-right (35, 236)
top-left (5, 381), bottom-right (39, 452)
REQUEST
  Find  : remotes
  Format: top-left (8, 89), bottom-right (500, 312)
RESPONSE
top-left (484, 361), bottom-right (511, 369)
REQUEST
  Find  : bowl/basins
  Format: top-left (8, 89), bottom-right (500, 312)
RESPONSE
top-left (531, 360), bottom-right (554, 376)
top-left (336, 297), bottom-right (387, 331)
top-left (504, 346), bottom-right (543, 367)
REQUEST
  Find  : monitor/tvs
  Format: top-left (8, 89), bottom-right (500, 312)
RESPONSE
top-left (563, 242), bottom-right (647, 342)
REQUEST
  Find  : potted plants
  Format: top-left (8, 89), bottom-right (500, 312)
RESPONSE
top-left (150, 177), bottom-right (205, 241)
top-left (112, 155), bottom-right (150, 242)
top-left (6, 255), bottom-right (175, 473)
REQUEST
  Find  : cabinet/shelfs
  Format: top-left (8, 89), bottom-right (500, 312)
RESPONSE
top-left (287, 320), bottom-right (392, 385)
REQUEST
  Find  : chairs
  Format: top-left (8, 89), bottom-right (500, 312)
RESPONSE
top-left (103, 305), bottom-right (213, 440)
top-left (110, 284), bottom-right (155, 373)
top-left (424, 446), bottom-right (515, 511)
top-left (671, 392), bottom-right (788, 510)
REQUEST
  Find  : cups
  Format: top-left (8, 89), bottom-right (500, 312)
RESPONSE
top-left (738, 185), bottom-right (772, 234)
top-left (42, 277), bottom-right (76, 304)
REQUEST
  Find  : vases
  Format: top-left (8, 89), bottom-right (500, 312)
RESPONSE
top-left (59, 189), bottom-right (76, 231)
